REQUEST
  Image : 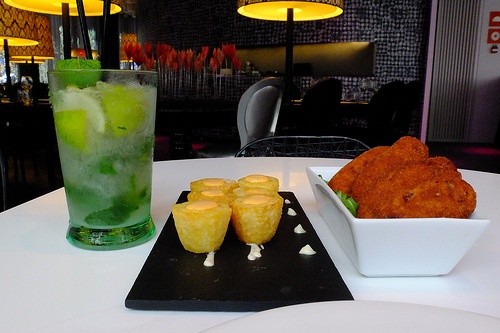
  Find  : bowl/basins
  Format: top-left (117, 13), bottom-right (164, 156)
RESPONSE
top-left (306, 165), bottom-right (492, 279)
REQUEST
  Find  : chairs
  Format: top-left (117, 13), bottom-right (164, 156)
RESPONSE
top-left (234, 73), bottom-right (421, 158)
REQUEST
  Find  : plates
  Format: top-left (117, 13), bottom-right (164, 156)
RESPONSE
top-left (201, 300), bottom-right (500, 333)
top-left (125, 191), bottom-right (355, 312)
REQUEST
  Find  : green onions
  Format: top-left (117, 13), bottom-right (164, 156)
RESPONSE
top-left (335, 191), bottom-right (358, 215)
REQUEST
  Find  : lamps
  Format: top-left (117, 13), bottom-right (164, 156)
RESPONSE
top-left (0, 0), bottom-right (128, 86)
top-left (236, 0), bottom-right (345, 96)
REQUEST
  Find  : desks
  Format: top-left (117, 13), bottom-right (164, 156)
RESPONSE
top-left (0, 156), bottom-right (500, 333)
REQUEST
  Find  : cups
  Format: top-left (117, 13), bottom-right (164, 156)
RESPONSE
top-left (17, 75), bottom-right (34, 106)
top-left (0, 85), bottom-right (11, 103)
top-left (47, 68), bottom-right (159, 251)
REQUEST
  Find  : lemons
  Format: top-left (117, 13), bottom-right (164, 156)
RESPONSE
top-left (56, 59), bottom-right (154, 226)
